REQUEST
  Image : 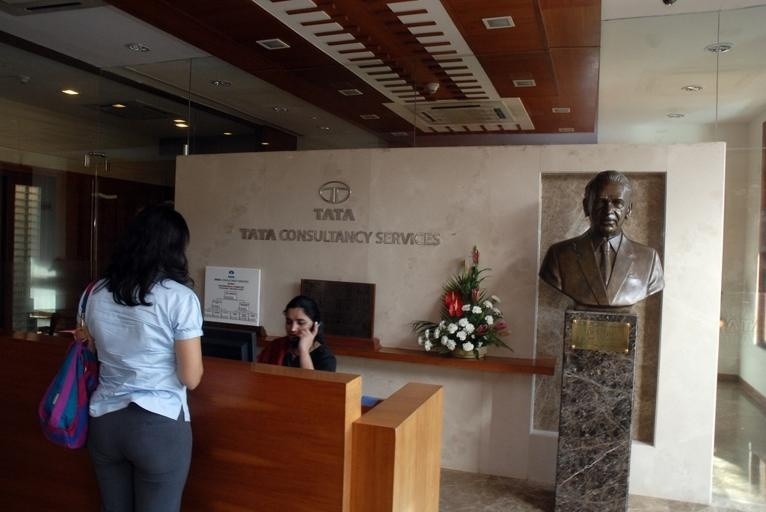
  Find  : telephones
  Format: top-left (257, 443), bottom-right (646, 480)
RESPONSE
top-left (290, 320), bottom-right (324, 347)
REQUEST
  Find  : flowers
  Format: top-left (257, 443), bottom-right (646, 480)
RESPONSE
top-left (411, 246), bottom-right (514, 360)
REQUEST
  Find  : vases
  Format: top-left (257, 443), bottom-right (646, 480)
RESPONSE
top-left (450, 345), bottom-right (488, 361)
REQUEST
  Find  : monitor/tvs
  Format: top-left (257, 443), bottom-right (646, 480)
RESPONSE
top-left (201, 326), bottom-right (256, 362)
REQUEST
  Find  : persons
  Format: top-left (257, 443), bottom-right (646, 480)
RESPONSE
top-left (254, 293), bottom-right (336, 372)
top-left (72, 205), bottom-right (203, 512)
top-left (537, 170), bottom-right (665, 307)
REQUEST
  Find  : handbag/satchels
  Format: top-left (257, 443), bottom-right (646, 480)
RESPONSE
top-left (38, 275), bottom-right (105, 450)
